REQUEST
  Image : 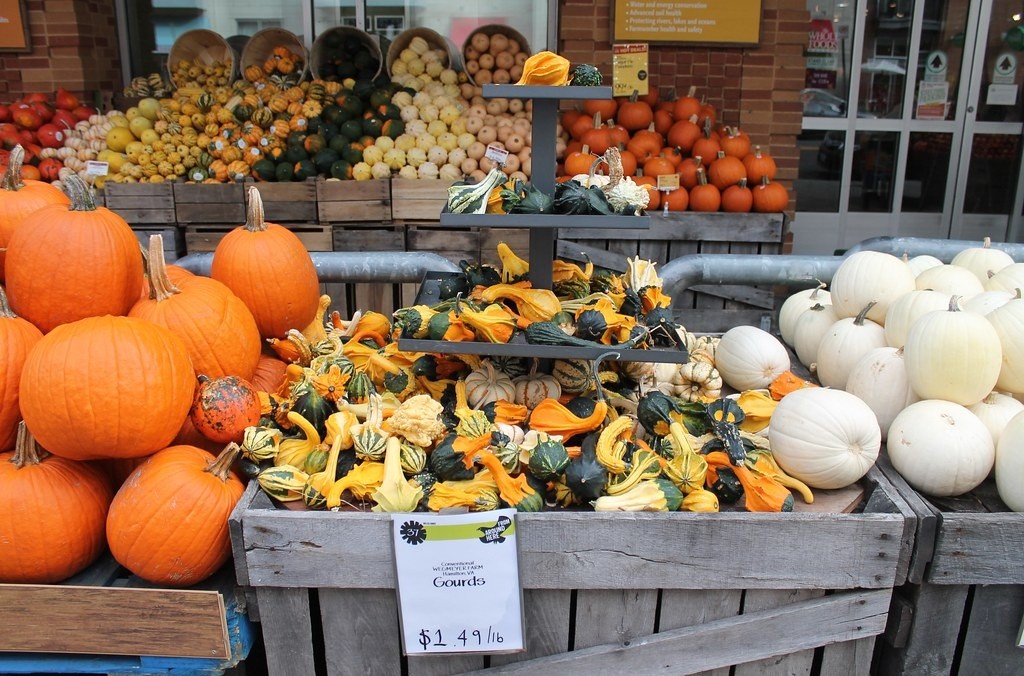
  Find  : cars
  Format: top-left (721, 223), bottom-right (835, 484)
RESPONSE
top-left (817, 114), bottom-right (899, 179)
top-left (798, 88), bottom-right (847, 139)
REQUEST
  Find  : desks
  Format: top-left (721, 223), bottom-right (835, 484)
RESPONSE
top-left (915, 133), bottom-right (1021, 214)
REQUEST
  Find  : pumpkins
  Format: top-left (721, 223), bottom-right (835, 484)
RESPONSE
top-left (0, 35), bottom-right (1024, 585)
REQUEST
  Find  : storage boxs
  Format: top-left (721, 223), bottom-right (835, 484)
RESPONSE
top-left (0, 167), bottom-right (1022, 675)
